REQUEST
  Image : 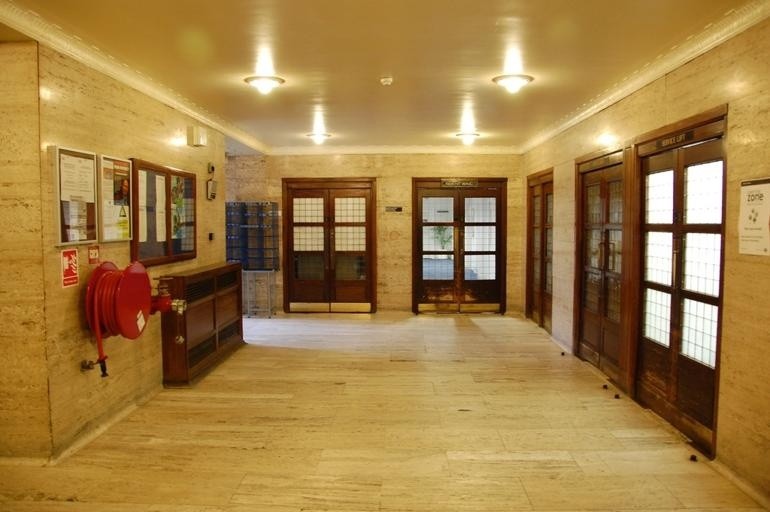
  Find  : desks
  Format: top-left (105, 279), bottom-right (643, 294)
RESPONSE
top-left (242, 269), bottom-right (277, 319)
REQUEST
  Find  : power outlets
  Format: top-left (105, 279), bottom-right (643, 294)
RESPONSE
top-left (208, 163), bottom-right (215, 173)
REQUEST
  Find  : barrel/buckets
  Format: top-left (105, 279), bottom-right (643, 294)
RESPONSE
top-left (227, 201), bottom-right (280, 270)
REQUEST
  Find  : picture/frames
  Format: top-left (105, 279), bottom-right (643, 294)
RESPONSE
top-left (129, 158), bottom-right (197, 267)
top-left (98, 154), bottom-right (133, 243)
top-left (53, 146), bottom-right (99, 247)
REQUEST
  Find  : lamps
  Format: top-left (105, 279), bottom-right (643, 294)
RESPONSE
top-left (307, 134), bottom-right (331, 144)
top-left (456, 134), bottom-right (480, 144)
top-left (193, 126), bottom-right (208, 147)
top-left (245, 76), bottom-right (285, 94)
top-left (492, 75), bottom-right (534, 93)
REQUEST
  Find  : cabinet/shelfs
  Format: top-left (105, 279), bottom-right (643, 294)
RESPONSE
top-left (160, 263), bottom-right (247, 389)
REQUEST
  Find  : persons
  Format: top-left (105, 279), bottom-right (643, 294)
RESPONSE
top-left (114, 180), bottom-right (129, 206)
